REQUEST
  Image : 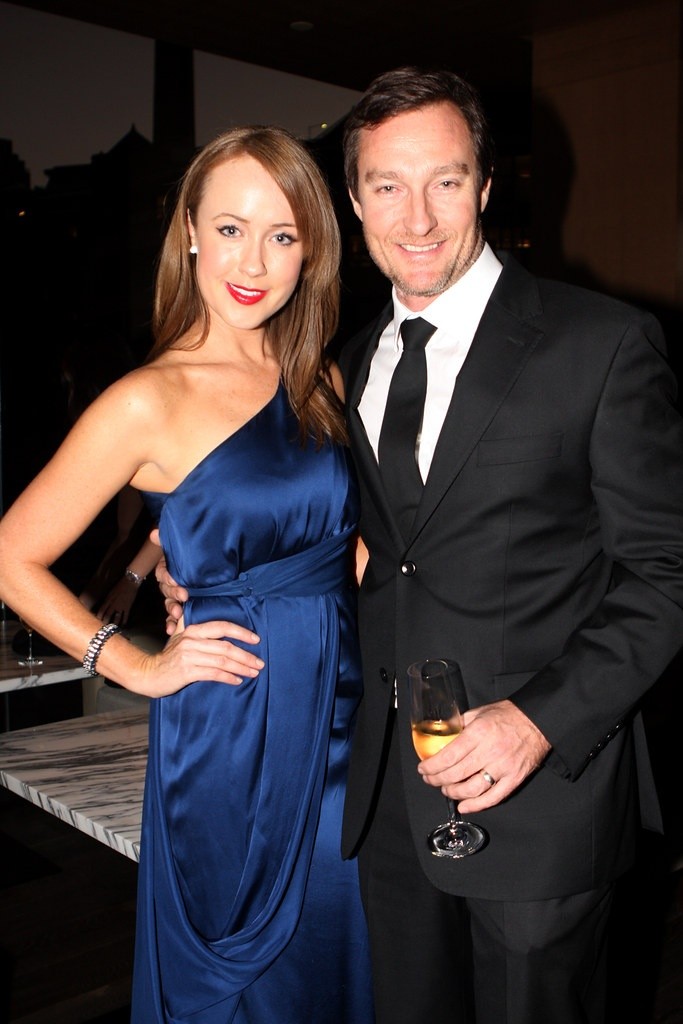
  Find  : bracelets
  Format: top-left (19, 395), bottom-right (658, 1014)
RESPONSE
top-left (81, 623), bottom-right (120, 676)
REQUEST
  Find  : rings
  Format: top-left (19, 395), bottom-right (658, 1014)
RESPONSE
top-left (481, 770), bottom-right (496, 786)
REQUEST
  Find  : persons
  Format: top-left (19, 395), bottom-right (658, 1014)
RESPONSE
top-left (3, 127), bottom-right (378, 1023)
top-left (328, 66), bottom-right (683, 1021)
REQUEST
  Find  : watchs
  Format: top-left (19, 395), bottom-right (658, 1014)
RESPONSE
top-left (125, 568), bottom-right (143, 586)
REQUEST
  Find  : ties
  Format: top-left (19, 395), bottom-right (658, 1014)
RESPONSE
top-left (377, 316), bottom-right (438, 538)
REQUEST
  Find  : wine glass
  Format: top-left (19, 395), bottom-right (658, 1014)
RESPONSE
top-left (18, 616), bottom-right (43, 666)
top-left (407, 659), bottom-right (485, 858)
